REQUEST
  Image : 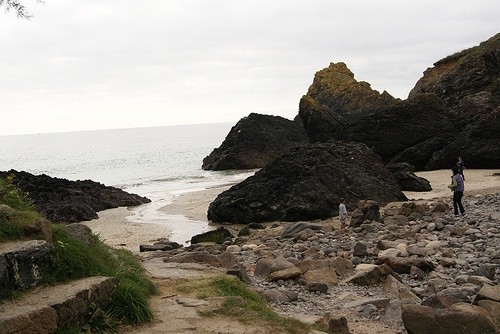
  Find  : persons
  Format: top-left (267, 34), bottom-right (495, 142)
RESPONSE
top-left (447, 165), bottom-right (466, 216)
top-left (337, 197), bottom-right (348, 228)
top-left (455, 157), bottom-right (466, 181)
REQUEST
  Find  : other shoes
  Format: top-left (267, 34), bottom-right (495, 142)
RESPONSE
top-left (453, 214), bottom-right (458, 217)
top-left (462, 213), bottom-right (468, 217)
